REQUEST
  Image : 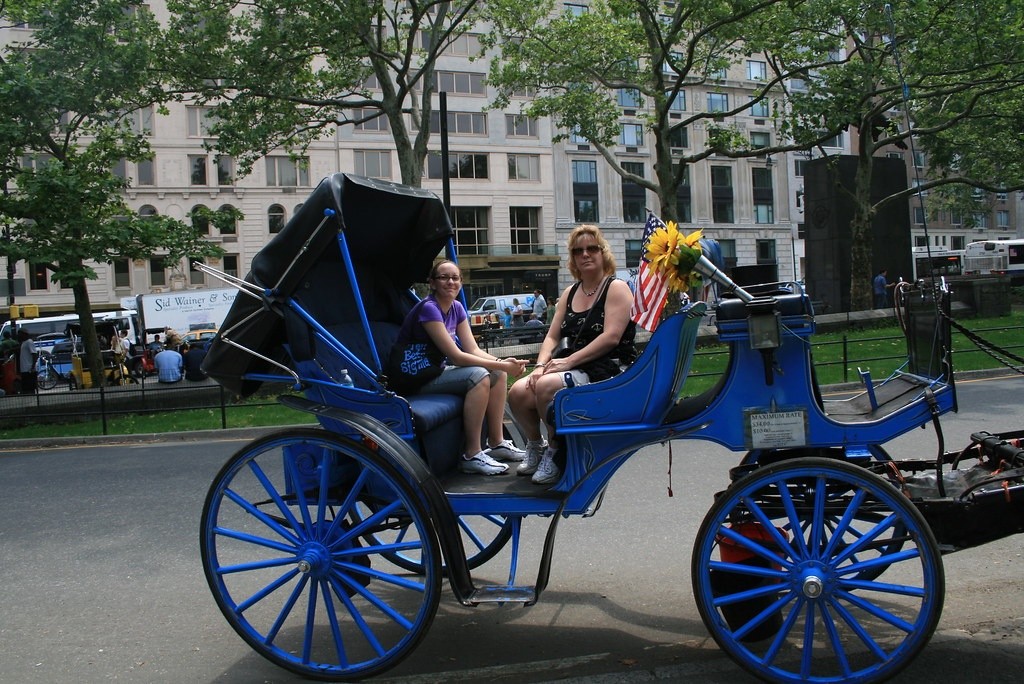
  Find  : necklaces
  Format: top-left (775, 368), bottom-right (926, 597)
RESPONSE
top-left (582, 274), bottom-right (605, 297)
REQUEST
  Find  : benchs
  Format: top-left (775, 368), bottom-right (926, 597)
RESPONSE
top-left (542, 299), bottom-right (708, 492)
top-left (283, 321), bottom-right (488, 485)
top-left (75, 351), bottom-right (117, 371)
top-left (482, 324), bottom-right (551, 349)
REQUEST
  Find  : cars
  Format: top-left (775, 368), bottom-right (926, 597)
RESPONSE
top-left (181, 329), bottom-right (218, 351)
top-left (50, 337), bottom-right (149, 377)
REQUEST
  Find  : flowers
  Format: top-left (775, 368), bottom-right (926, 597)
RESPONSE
top-left (644, 221), bottom-right (705, 291)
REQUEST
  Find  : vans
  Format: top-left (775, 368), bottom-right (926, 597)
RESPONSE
top-left (468, 293), bottom-right (547, 334)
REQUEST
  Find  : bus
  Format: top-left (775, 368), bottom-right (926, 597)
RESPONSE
top-left (0, 310), bottom-right (137, 381)
top-left (912, 239), bottom-right (1024, 286)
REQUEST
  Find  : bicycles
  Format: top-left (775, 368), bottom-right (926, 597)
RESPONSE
top-left (37, 355), bottom-right (58, 389)
top-left (482, 312), bottom-right (494, 348)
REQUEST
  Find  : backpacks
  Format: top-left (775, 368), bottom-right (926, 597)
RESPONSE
top-left (127, 339), bottom-right (137, 356)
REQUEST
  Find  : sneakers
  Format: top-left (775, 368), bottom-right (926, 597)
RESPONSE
top-left (458, 449), bottom-right (510, 476)
top-left (532, 450), bottom-right (561, 483)
top-left (486, 438), bottom-right (526, 461)
top-left (517, 440), bottom-right (549, 475)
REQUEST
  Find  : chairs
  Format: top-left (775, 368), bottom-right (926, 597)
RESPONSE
top-left (698, 237), bottom-right (817, 338)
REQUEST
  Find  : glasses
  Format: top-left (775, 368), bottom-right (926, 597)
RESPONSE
top-left (572, 246), bottom-right (601, 255)
top-left (434, 274), bottom-right (461, 282)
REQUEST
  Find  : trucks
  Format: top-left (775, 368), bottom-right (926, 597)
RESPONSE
top-left (136, 287), bottom-right (239, 350)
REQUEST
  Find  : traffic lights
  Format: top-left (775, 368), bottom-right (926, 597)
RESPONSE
top-left (9, 305), bottom-right (20, 320)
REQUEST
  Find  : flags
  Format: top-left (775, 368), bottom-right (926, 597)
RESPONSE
top-left (629, 213), bottom-right (677, 333)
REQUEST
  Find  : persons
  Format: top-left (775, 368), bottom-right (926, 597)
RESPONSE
top-left (111, 328), bottom-right (134, 385)
top-left (533, 289), bottom-right (547, 324)
top-left (164, 324), bottom-right (188, 376)
top-left (874, 268), bottom-right (896, 310)
top-left (147, 335), bottom-right (164, 360)
top-left (387, 261), bottom-right (526, 475)
top-left (0, 327), bottom-right (38, 395)
top-left (547, 296), bottom-right (560, 324)
top-left (524, 312), bottom-right (544, 327)
top-left (508, 223), bottom-right (637, 484)
top-left (511, 297), bottom-right (525, 328)
top-left (183, 343), bottom-right (209, 381)
top-left (154, 340), bottom-right (184, 384)
top-left (680, 291), bottom-right (690, 307)
top-left (502, 307), bottom-right (512, 329)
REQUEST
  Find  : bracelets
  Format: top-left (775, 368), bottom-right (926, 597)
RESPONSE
top-left (535, 362), bottom-right (545, 368)
top-left (495, 358), bottom-right (503, 361)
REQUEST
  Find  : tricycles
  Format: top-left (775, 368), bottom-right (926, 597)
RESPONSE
top-left (140, 327), bottom-right (180, 376)
top-left (64, 317), bottom-right (139, 390)
